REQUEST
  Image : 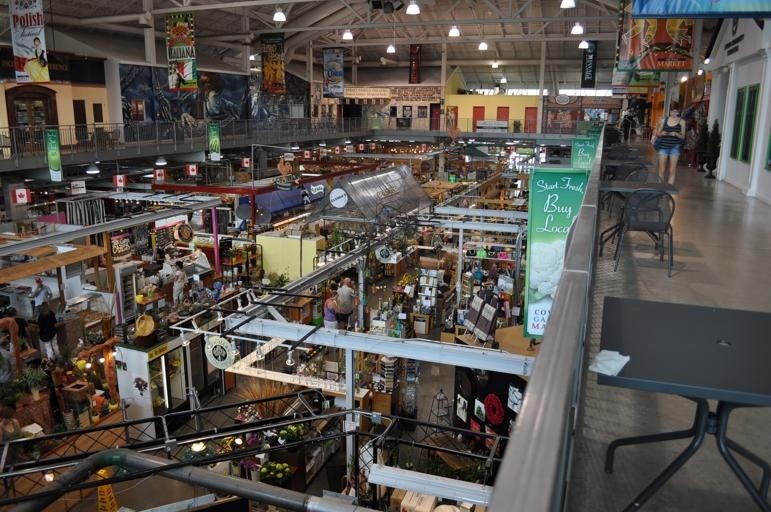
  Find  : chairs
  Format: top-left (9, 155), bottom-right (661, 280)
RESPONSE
top-left (599, 127), bottom-right (679, 278)
top-left (0, 129), bottom-right (122, 157)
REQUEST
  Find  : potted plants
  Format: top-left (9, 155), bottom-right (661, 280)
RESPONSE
top-left (696, 119), bottom-right (711, 172)
top-left (704, 119), bottom-right (721, 179)
top-left (15, 364), bottom-right (48, 401)
top-left (108, 398), bottom-right (118, 410)
top-left (90, 410), bottom-right (100, 422)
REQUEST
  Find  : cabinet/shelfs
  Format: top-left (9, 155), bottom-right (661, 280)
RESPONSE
top-left (221, 251), bottom-right (248, 287)
top-left (239, 244), bottom-right (263, 281)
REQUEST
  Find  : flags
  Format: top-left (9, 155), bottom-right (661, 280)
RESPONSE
top-left (12, 157), bottom-right (252, 203)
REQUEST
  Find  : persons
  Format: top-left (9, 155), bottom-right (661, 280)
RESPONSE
top-left (321, 274), bottom-right (359, 330)
top-left (171, 260), bottom-right (187, 305)
top-left (163, 246), bottom-right (176, 262)
top-left (180, 71), bottom-right (251, 127)
top-left (23, 36), bottom-right (48, 83)
top-left (6, 306), bottom-right (32, 353)
top-left (36, 301), bottom-right (63, 362)
top-left (656, 101), bottom-right (687, 189)
top-left (620, 109), bottom-right (643, 139)
top-left (30, 276), bottom-right (53, 320)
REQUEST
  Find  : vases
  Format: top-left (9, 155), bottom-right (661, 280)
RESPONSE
top-left (148, 291), bottom-right (155, 298)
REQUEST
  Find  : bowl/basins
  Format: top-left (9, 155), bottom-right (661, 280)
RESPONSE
top-left (136, 314), bottom-right (155, 337)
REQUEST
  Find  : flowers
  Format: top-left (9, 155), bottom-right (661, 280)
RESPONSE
top-left (140, 284), bottom-right (157, 294)
top-left (182, 402), bottom-right (307, 479)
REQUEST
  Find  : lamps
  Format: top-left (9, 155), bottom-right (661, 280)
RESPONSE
top-left (273, 3), bottom-right (287, 22)
top-left (578, 40), bottom-right (589, 49)
top-left (168, 288), bottom-right (313, 366)
top-left (405, 0), bottom-right (421, 16)
top-left (155, 156), bottom-right (168, 167)
top-left (342, 29), bottom-right (353, 41)
top-left (570, 21), bottom-right (584, 35)
top-left (386, 45), bottom-right (396, 53)
top-left (86, 164), bottom-right (101, 174)
top-left (559, 0), bottom-right (576, 9)
top-left (478, 42), bottom-right (488, 51)
top-left (447, 25), bottom-right (460, 38)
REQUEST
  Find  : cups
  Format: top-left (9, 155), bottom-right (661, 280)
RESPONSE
top-left (136, 294), bottom-right (144, 304)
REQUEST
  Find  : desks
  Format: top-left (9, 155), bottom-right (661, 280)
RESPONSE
top-left (595, 296), bottom-right (771, 511)
